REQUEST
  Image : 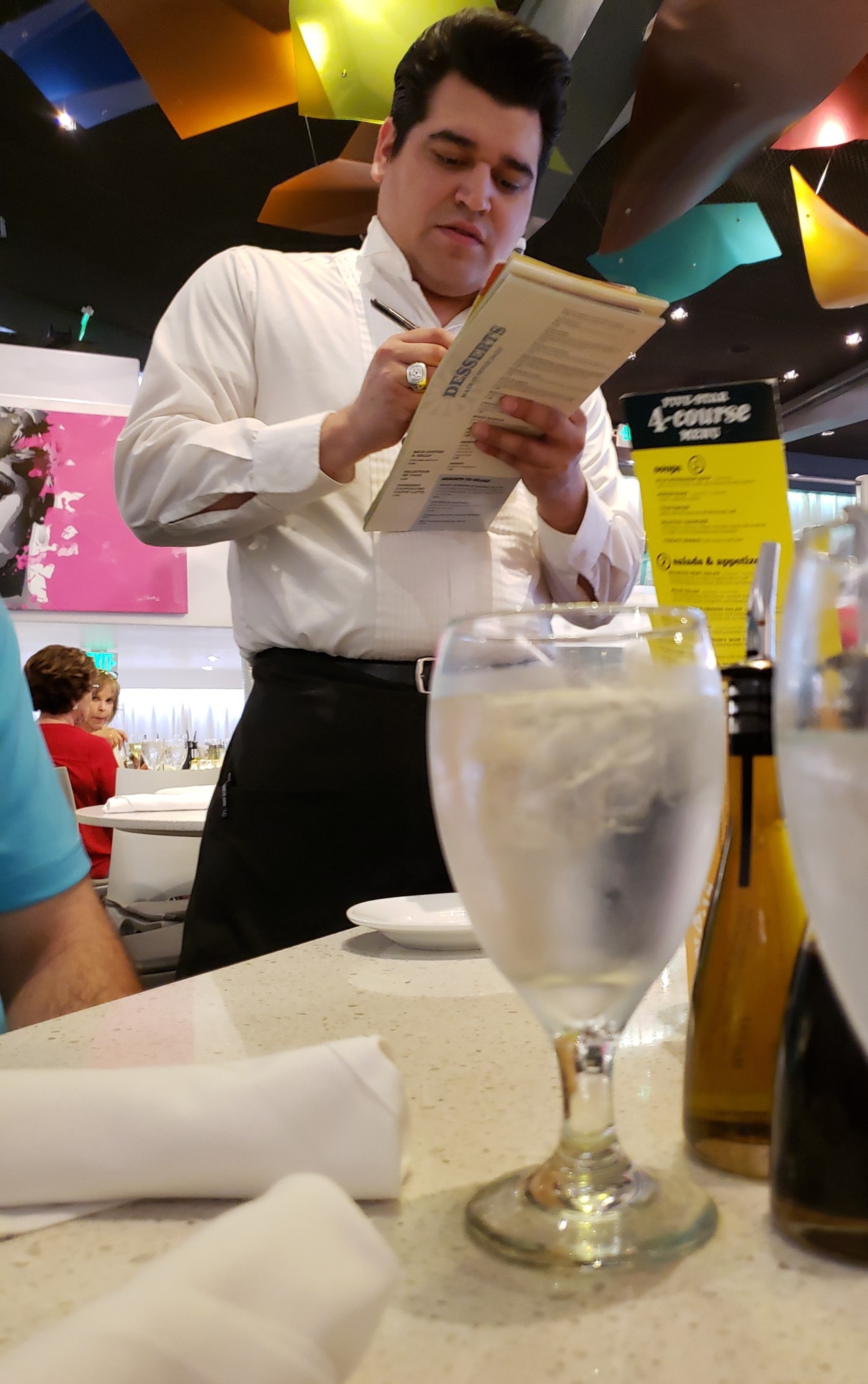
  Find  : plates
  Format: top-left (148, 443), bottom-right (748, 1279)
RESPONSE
top-left (344, 891), bottom-right (482, 951)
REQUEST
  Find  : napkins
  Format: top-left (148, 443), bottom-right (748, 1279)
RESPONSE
top-left (0, 1029), bottom-right (414, 1239)
top-left (99, 791), bottom-right (210, 814)
top-left (0, 1166), bottom-right (397, 1384)
top-left (155, 783), bottom-right (216, 796)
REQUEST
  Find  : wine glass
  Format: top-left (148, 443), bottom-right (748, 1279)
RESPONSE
top-left (424, 605), bottom-right (732, 1284)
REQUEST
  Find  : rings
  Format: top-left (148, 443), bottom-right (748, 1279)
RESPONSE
top-left (405, 361), bottom-right (428, 393)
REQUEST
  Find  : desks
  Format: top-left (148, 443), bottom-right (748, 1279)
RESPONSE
top-left (72, 778), bottom-right (216, 839)
top-left (0, 918), bottom-right (868, 1384)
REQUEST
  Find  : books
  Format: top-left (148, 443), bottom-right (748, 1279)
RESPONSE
top-left (365, 246), bottom-right (668, 538)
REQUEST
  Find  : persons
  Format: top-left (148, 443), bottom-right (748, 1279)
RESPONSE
top-left (1, 596), bottom-right (139, 1030)
top-left (110, 10), bottom-right (649, 979)
top-left (75, 669), bottom-right (147, 770)
top-left (26, 644), bottom-right (120, 880)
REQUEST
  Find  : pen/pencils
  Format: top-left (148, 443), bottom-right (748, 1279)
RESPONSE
top-left (371, 299), bottom-right (418, 331)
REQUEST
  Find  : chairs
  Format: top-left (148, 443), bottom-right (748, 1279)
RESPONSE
top-left (51, 763), bottom-right (222, 995)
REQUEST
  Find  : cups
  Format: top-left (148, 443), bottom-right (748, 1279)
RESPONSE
top-left (767, 520), bottom-right (868, 1054)
top-left (128, 735), bottom-right (189, 771)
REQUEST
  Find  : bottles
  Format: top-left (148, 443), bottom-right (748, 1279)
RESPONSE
top-left (764, 921), bottom-right (867, 1273)
top-left (680, 664), bottom-right (808, 1183)
top-left (183, 737), bottom-right (232, 770)
